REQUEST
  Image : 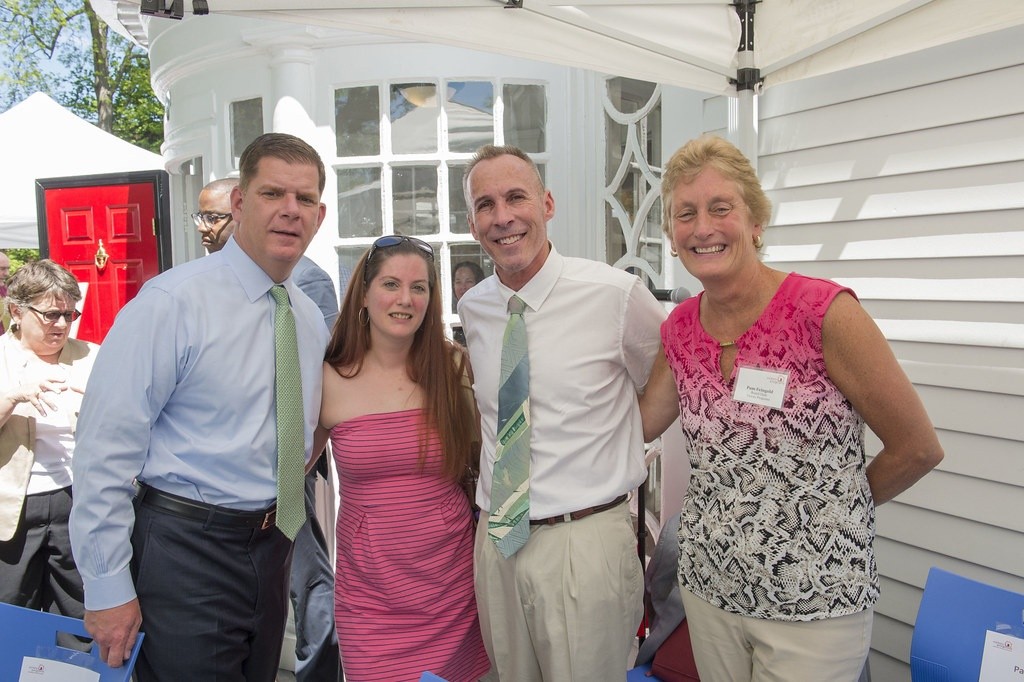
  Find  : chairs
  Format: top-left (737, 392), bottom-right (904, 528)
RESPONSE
top-left (0, 602), bottom-right (146, 682)
top-left (909, 567), bottom-right (1024, 682)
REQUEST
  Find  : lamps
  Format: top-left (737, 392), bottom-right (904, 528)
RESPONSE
top-left (393, 82), bottom-right (465, 108)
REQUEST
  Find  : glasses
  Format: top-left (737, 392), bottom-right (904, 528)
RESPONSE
top-left (192, 213), bottom-right (231, 226)
top-left (365, 234), bottom-right (435, 265)
top-left (24, 304), bottom-right (81, 322)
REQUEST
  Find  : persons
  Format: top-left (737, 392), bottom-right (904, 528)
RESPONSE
top-left (68, 133), bottom-right (945, 682)
top-left (0, 251), bottom-right (101, 655)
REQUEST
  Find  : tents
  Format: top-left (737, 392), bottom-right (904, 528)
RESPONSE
top-left (0, 91), bottom-right (165, 250)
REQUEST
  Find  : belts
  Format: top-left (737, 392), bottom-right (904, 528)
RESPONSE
top-left (529, 494), bottom-right (627, 525)
top-left (132, 479), bottom-right (276, 532)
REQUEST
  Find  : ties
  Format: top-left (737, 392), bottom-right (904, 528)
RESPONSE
top-left (269, 286), bottom-right (307, 542)
top-left (487, 296), bottom-right (531, 563)
